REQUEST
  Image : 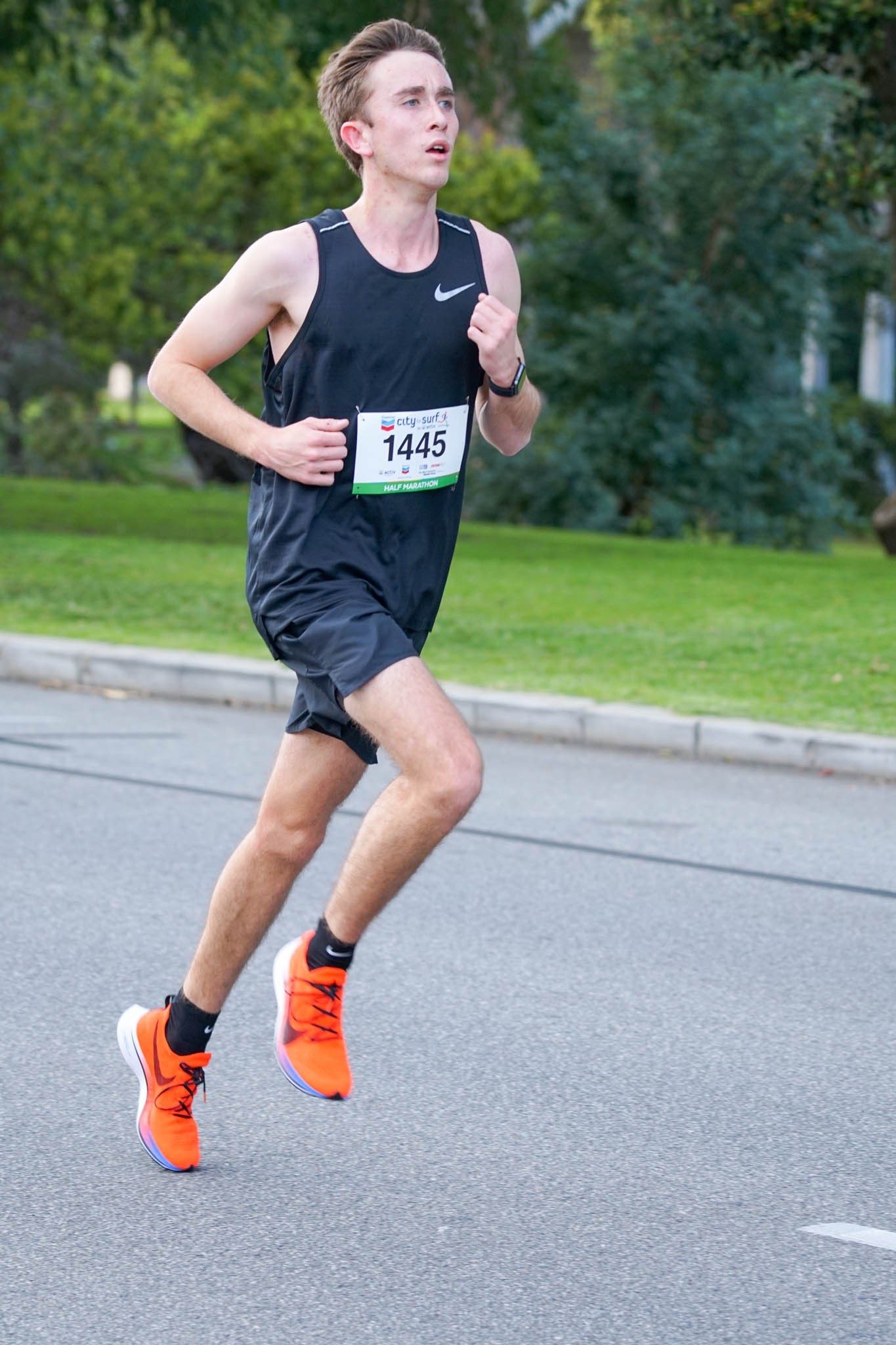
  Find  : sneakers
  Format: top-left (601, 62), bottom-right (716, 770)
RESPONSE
top-left (116, 993), bottom-right (211, 1171)
top-left (273, 929), bottom-right (351, 1102)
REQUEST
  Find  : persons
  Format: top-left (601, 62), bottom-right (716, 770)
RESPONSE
top-left (113, 21), bottom-right (542, 1173)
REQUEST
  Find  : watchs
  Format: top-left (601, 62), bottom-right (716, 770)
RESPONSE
top-left (489, 358), bottom-right (527, 398)
